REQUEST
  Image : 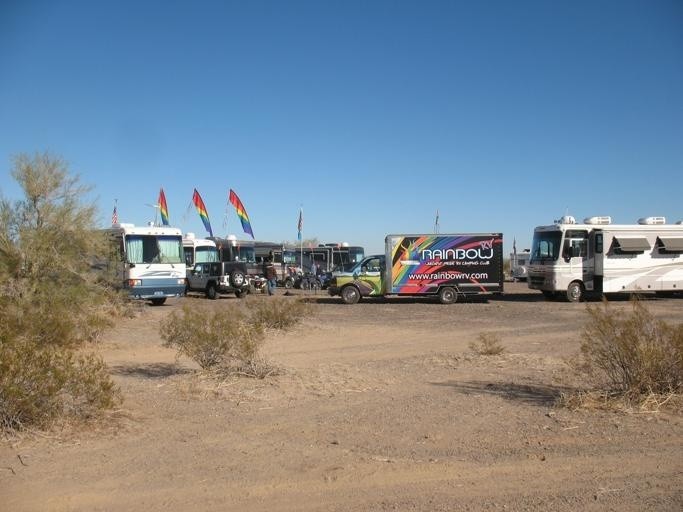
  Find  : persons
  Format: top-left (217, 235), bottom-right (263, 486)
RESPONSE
top-left (262, 262), bottom-right (277, 296)
top-left (315, 263), bottom-right (321, 279)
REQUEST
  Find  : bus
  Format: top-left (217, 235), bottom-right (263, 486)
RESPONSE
top-left (527, 217), bottom-right (683, 302)
top-left (510, 252), bottom-right (529, 282)
top-left (19, 223), bottom-right (187, 305)
top-left (183, 235), bottom-right (363, 289)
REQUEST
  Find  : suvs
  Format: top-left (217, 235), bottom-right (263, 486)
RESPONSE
top-left (187, 262), bottom-right (250, 298)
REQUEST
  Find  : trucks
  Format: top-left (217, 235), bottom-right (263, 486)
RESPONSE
top-left (329, 234), bottom-right (503, 304)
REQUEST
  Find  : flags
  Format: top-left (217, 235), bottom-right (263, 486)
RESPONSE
top-left (110, 206), bottom-right (118, 224)
top-left (192, 189), bottom-right (213, 238)
top-left (513, 236), bottom-right (516, 256)
top-left (297, 207), bottom-right (303, 232)
top-left (435, 209), bottom-right (439, 225)
top-left (157, 188), bottom-right (169, 225)
top-left (229, 188), bottom-right (255, 240)
